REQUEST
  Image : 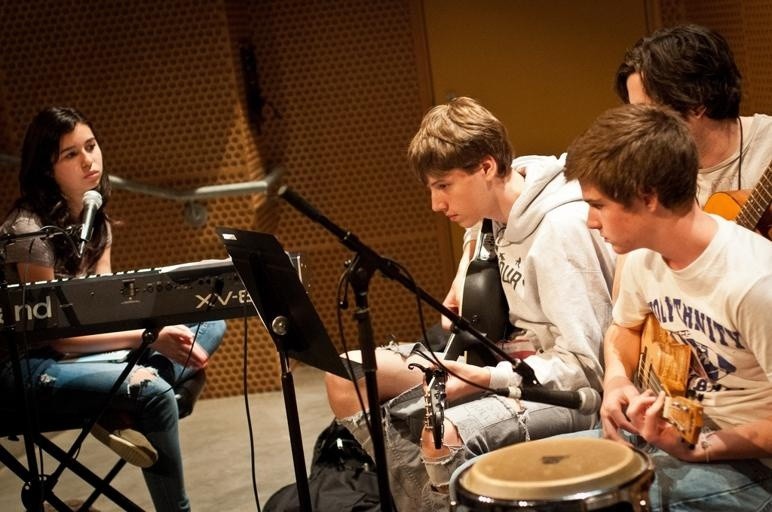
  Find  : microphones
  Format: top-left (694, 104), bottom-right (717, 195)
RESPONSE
top-left (79, 190), bottom-right (103, 256)
top-left (496, 386), bottom-right (602, 417)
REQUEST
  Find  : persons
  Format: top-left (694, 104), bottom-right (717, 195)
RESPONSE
top-left (1, 104), bottom-right (227, 512)
top-left (609, 22), bottom-right (771, 209)
top-left (323, 94), bottom-right (616, 511)
top-left (435, 103), bottom-right (772, 512)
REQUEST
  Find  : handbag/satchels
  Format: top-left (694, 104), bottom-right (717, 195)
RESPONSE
top-left (258, 419), bottom-right (384, 512)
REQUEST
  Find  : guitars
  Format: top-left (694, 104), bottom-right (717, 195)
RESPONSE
top-left (631, 312), bottom-right (704, 451)
top-left (702, 158), bottom-right (772, 243)
top-left (421, 217), bottom-right (509, 450)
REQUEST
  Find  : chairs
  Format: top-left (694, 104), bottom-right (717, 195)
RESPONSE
top-left (0, 370), bottom-right (206, 512)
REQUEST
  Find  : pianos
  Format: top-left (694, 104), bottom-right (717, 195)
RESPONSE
top-left (3, 246), bottom-right (304, 351)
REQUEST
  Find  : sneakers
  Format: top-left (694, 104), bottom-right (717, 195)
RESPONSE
top-left (92, 418), bottom-right (159, 467)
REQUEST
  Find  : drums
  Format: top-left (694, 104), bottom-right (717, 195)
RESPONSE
top-left (457, 437), bottom-right (654, 512)
